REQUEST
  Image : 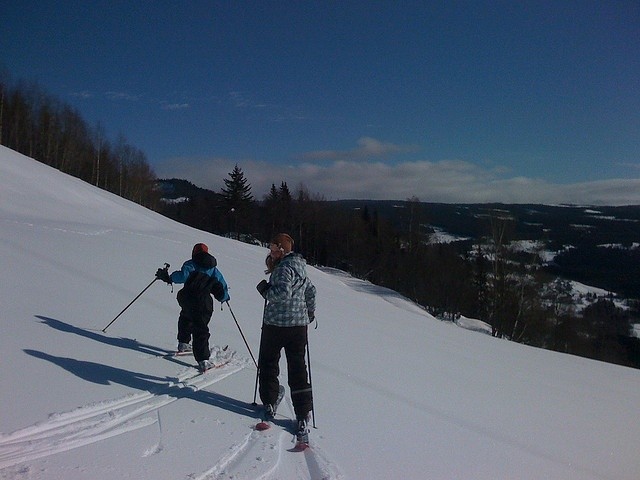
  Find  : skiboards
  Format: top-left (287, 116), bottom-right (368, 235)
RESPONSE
top-left (255, 384), bottom-right (313, 453)
top-left (154, 343), bottom-right (233, 380)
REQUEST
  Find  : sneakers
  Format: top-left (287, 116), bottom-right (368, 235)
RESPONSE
top-left (198, 360), bottom-right (215, 371)
top-left (265, 403), bottom-right (277, 416)
top-left (299, 414), bottom-right (311, 434)
top-left (177, 343), bottom-right (192, 351)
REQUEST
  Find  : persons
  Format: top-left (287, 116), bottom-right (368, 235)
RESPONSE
top-left (156, 243), bottom-right (231, 370)
top-left (255, 233), bottom-right (316, 429)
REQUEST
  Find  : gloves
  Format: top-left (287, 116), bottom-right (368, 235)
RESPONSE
top-left (256, 279), bottom-right (271, 299)
top-left (155, 268), bottom-right (172, 285)
top-left (308, 311), bottom-right (314, 323)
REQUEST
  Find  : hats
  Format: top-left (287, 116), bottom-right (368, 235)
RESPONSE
top-left (270, 234), bottom-right (294, 256)
top-left (193, 243), bottom-right (208, 254)
